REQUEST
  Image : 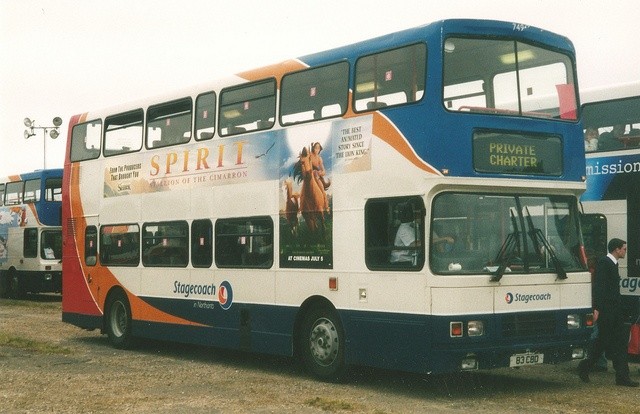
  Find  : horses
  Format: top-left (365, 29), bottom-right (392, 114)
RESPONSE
top-left (294, 146), bottom-right (331, 233)
top-left (284, 179), bottom-right (301, 233)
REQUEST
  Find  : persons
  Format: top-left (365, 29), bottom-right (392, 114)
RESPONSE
top-left (599, 121), bottom-right (626, 150)
top-left (309, 141), bottom-right (327, 177)
top-left (583, 126), bottom-right (600, 153)
top-left (388, 213), bottom-right (454, 265)
top-left (575, 237), bottom-right (639, 387)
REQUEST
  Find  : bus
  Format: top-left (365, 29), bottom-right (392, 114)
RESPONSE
top-left (62, 18), bottom-right (598, 378)
top-left (504, 84), bottom-right (640, 358)
top-left (0, 168), bottom-right (63, 298)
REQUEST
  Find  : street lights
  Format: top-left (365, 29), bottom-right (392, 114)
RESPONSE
top-left (24, 117), bottom-right (62, 169)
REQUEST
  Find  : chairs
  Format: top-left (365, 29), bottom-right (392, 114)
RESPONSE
top-left (107, 231), bottom-right (153, 256)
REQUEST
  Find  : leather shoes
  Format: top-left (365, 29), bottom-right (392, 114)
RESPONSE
top-left (576, 365), bottom-right (590, 383)
top-left (615, 376), bottom-right (640, 387)
